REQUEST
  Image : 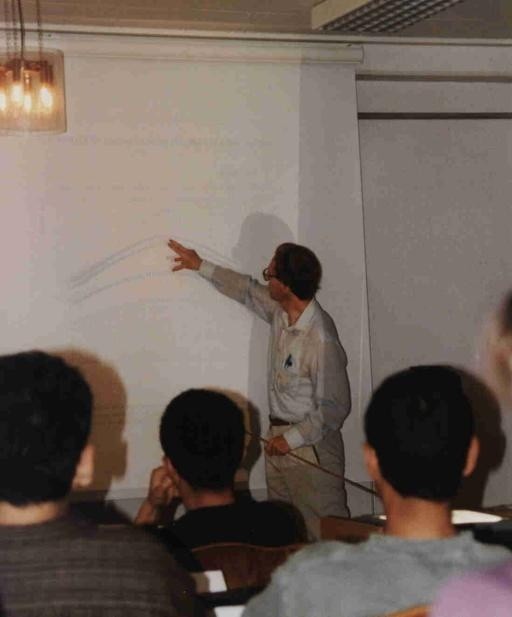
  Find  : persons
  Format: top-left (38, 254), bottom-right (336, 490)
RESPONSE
top-left (0, 351), bottom-right (199, 617)
top-left (134, 387), bottom-right (308, 617)
top-left (242, 361), bottom-right (512, 617)
top-left (168, 238), bottom-right (353, 550)
top-left (424, 286), bottom-right (512, 617)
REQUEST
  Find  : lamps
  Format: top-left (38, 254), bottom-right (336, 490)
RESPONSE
top-left (0, 1), bottom-right (67, 136)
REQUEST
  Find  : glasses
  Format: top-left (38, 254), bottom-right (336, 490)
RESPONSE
top-left (263, 268), bottom-right (279, 281)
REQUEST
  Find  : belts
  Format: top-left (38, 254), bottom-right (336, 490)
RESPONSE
top-left (268, 414), bottom-right (296, 425)
